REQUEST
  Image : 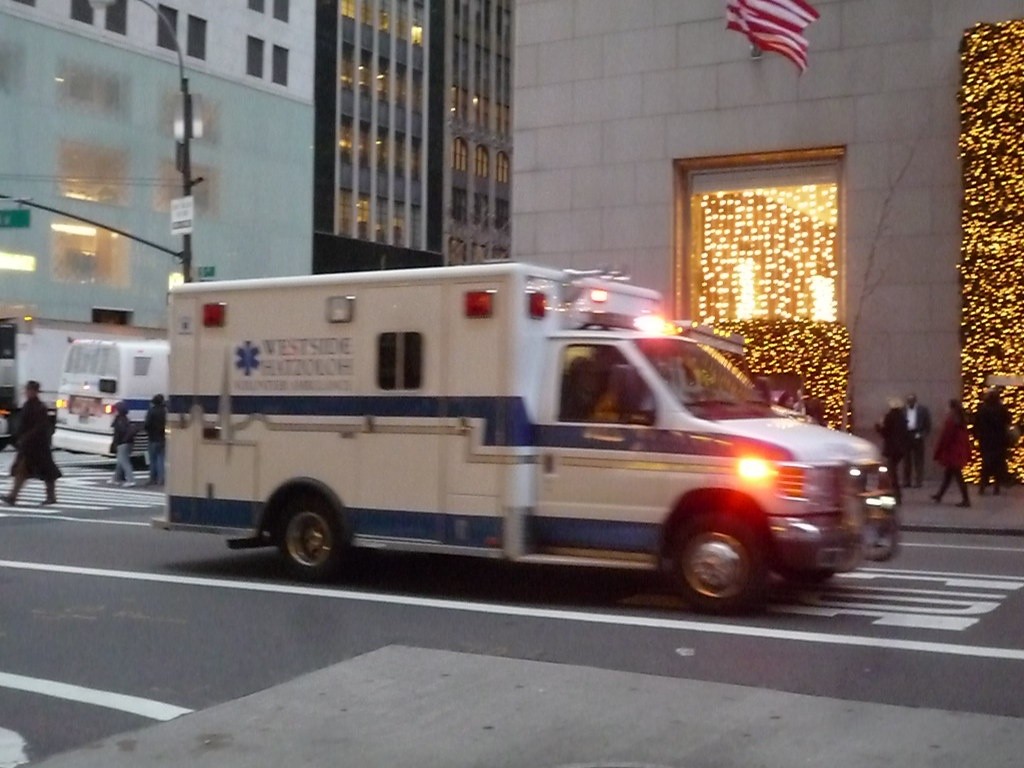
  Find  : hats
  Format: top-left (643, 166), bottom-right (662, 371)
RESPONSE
top-left (25, 380), bottom-right (44, 394)
top-left (152, 394), bottom-right (164, 403)
top-left (115, 402), bottom-right (128, 415)
top-left (888, 397), bottom-right (903, 408)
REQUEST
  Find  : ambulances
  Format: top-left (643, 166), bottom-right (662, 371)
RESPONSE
top-left (151, 260), bottom-right (892, 618)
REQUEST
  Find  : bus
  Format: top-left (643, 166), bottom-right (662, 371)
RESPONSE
top-left (1, 322), bottom-right (19, 448)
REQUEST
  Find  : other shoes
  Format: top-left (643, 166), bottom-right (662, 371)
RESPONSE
top-left (41, 497), bottom-right (56, 505)
top-left (913, 483), bottom-right (922, 488)
top-left (157, 482), bottom-right (162, 486)
top-left (897, 499), bottom-right (901, 504)
top-left (0, 496), bottom-right (15, 506)
top-left (145, 480), bottom-right (157, 485)
top-left (901, 483), bottom-right (911, 487)
top-left (122, 482), bottom-right (136, 487)
top-left (930, 494), bottom-right (941, 503)
top-left (979, 488), bottom-right (984, 494)
top-left (955, 500), bottom-right (970, 507)
top-left (107, 479), bottom-right (120, 486)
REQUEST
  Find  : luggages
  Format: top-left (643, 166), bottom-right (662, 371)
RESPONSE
top-left (992, 489), bottom-right (1000, 495)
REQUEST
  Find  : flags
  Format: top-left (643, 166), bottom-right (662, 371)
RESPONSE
top-left (725, 0), bottom-right (820, 79)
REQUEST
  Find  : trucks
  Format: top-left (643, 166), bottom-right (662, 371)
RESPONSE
top-left (51, 339), bottom-right (170, 472)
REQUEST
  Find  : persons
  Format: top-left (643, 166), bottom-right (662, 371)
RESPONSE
top-left (967, 391), bottom-right (1011, 497)
top-left (142, 394), bottom-right (165, 486)
top-left (0, 380), bottom-right (63, 506)
top-left (107, 402), bottom-right (137, 488)
top-left (898, 393), bottom-right (932, 487)
top-left (928, 398), bottom-right (972, 509)
top-left (873, 395), bottom-right (906, 506)
